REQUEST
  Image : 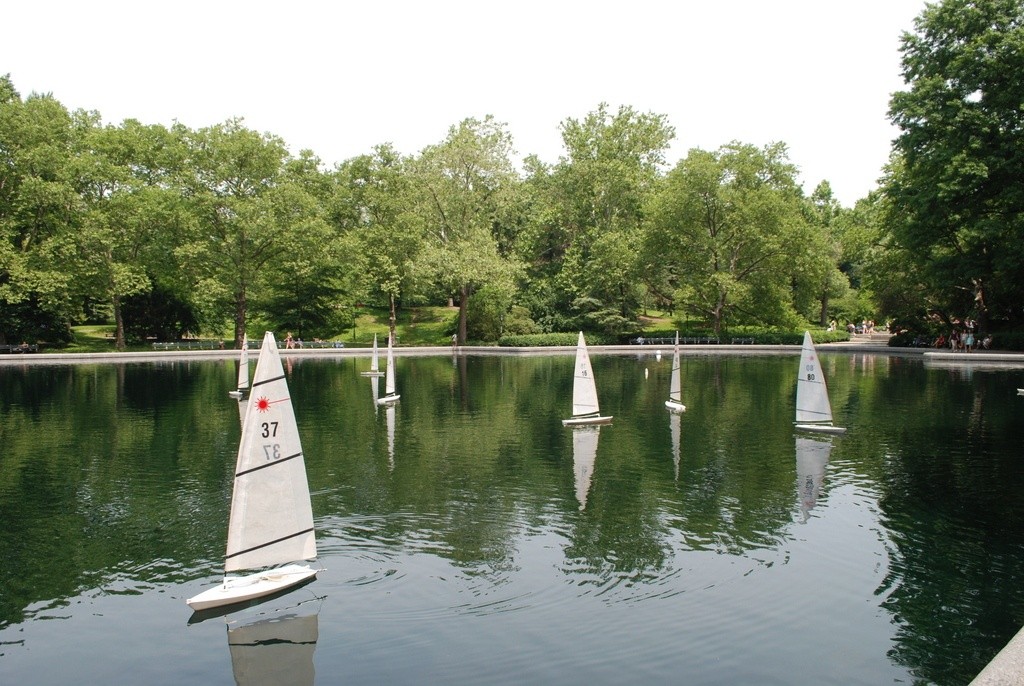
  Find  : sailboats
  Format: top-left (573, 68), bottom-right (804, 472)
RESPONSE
top-left (792, 330), bottom-right (848, 434)
top-left (360, 333), bottom-right (385, 375)
top-left (228, 331), bottom-right (250, 396)
top-left (664, 330), bottom-right (686, 411)
top-left (185, 331), bottom-right (328, 612)
top-left (560, 332), bottom-right (614, 426)
top-left (376, 332), bottom-right (401, 403)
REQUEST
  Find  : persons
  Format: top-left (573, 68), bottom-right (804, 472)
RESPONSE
top-left (19, 341), bottom-right (29, 353)
top-left (219, 340), bottom-right (226, 347)
top-left (451, 332), bottom-right (457, 347)
top-left (830, 314), bottom-right (992, 354)
top-left (335, 338), bottom-right (345, 348)
top-left (284, 331), bottom-right (304, 349)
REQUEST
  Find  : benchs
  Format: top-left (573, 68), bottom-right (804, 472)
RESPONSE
top-left (732, 338), bottom-right (754, 345)
top-left (643, 337), bottom-right (720, 344)
top-left (152, 340), bottom-right (218, 350)
top-left (248, 341), bottom-right (335, 348)
